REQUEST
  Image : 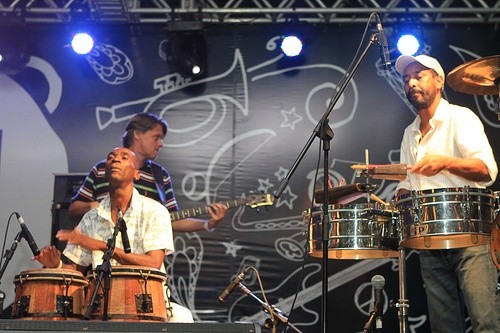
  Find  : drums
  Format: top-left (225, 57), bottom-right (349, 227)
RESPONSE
top-left (9, 268), bottom-right (89, 321)
top-left (85, 265), bottom-right (169, 322)
top-left (307, 204), bottom-right (401, 260)
top-left (394, 186), bottom-right (496, 251)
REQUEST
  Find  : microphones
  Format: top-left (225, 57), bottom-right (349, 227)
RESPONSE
top-left (371, 276), bottom-right (385, 313)
top-left (16, 213), bottom-right (41, 255)
top-left (376, 18), bottom-right (391, 70)
top-left (117, 209), bottom-right (131, 253)
top-left (217, 273), bottom-right (245, 304)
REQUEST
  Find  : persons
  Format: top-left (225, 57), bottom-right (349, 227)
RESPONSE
top-left (32, 147), bottom-right (175, 321)
top-left (69, 113), bottom-right (228, 232)
top-left (395, 54), bottom-right (500, 333)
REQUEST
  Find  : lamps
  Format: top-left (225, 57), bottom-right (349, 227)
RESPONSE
top-left (396, 17), bottom-right (423, 55)
top-left (67, 23), bottom-right (96, 55)
top-left (278, 12), bottom-right (303, 56)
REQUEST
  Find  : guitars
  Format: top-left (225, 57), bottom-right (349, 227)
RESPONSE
top-left (169, 189), bottom-right (276, 221)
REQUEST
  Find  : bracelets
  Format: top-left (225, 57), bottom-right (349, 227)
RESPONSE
top-left (57, 260), bottom-right (62, 268)
top-left (204, 221), bottom-right (215, 232)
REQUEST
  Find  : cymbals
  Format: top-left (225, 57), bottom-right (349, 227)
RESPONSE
top-left (446, 54), bottom-right (500, 95)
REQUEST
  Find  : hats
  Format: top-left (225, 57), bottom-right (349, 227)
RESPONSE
top-left (395, 54), bottom-right (445, 80)
